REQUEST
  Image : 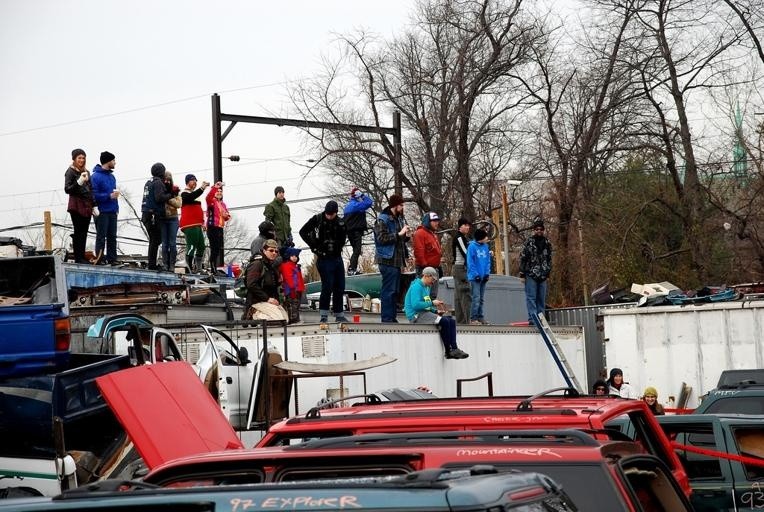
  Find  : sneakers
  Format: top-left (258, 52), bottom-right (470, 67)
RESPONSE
top-left (445, 348), bottom-right (469, 359)
top-left (108, 261), bottom-right (124, 268)
top-left (320, 314), bottom-right (328, 324)
top-left (95, 260), bottom-right (112, 266)
top-left (75, 259), bottom-right (93, 264)
top-left (148, 265), bottom-right (163, 272)
top-left (470, 319), bottom-right (482, 325)
top-left (290, 321), bottom-right (303, 326)
top-left (478, 320), bottom-right (491, 325)
top-left (347, 268), bottom-right (363, 276)
top-left (335, 317), bottom-right (351, 323)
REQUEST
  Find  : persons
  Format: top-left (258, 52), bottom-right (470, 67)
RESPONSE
top-left (453, 218), bottom-right (472, 325)
top-left (610, 369), bottom-right (629, 399)
top-left (90, 152), bottom-right (121, 264)
top-left (641, 389), bottom-right (667, 416)
top-left (345, 188), bottom-right (372, 274)
top-left (142, 162), bottom-right (180, 270)
top-left (282, 247), bottom-right (307, 322)
top-left (414, 211), bottom-right (442, 299)
top-left (520, 220), bottom-right (553, 325)
top-left (372, 195), bottom-right (411, 324)
top-left (242, 240), bottom-right (286, 321)
top-left (180, 173), bottom-right (210, 273)
top-left (251, 221), bottom-right (276, 254)
top-left (594, 377), bottom-right (606, 397)
top-left (467, 230), bottom-right (490, 324)
top-left (264, 186), bottom-right (294, 246)
top-left (299, 200), bottom-right (350, 324)
top-left (162, 172), bottom-right (182, 272)
top-left (404, 267), bottom-right (469, 358)
top-left (205, 181), bottom-right (231, 274)
top-left (64, 149), bottom-right (100, 264)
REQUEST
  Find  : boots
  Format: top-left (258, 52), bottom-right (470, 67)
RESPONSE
top-left (192, 254), bottom-right (203, 273)
top-left (162, 252), bottom-right (169, 271)
top-left (169, 252), bottom-right (177, 271)
top-left (185, 254), bottom-right (194, 274)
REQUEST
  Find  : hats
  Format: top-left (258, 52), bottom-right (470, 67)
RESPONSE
top-left (430, 211), bottom-right (442, 221)
top-left (72, 149), bottom-right (86, 160)
top-left (644, 388), bottom-right (658, 400)
top-left (325, 201), bottom-right (338, 212)
top-left (186, 175), bottom-right (197, 184)
top-left (165, 172), bottom-right (172, 179)
top-left (422, 267), bottom-right (437, 280)
top-left (262, 239), bottom-right (278, 248)
top-left (458, 217), bottom-right (472, 228)
top-left (275, 187), bottom-right (284, 194)
top-left (390, 195), bottom-right (405, 207)
top-left (285, 248), bottom-right (301, 262)
top-left (151, 162), bottom-right (165, 177)
top-left (475, 229), bottom-right (487, 241)
top-left (534, 220), bottom-right (545, 229)
top-left (100, 152), bottom-right (115, 164)
top-left (351, 188), bottom-right (359, 198)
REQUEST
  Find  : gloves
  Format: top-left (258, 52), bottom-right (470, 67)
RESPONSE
top-left (475, 276), bottom-right (480, 282)
top-left (483, 274), bottom-right (489, 283)
top-left (77, 171), bottom-right (89, 185)
top-left (91, 206), bottom-right (100, 216)
top-left (355, 190), bottom-right (363, 197)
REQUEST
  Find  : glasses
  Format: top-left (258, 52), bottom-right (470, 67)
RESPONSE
top-left (646, 396), bottom-right (656, 398)
top-left (266, 249), bottom-right (280, 253)
top-left (596, 388), bottom-right (605, 391)
top-left (536, 228), bottom-right (544, 231)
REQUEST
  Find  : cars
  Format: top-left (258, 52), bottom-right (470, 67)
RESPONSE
top-left (301, 274), bottom-right (383, 314)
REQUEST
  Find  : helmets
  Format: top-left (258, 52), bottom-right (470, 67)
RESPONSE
top-left (259, 221), bottom-right (276, 239)
